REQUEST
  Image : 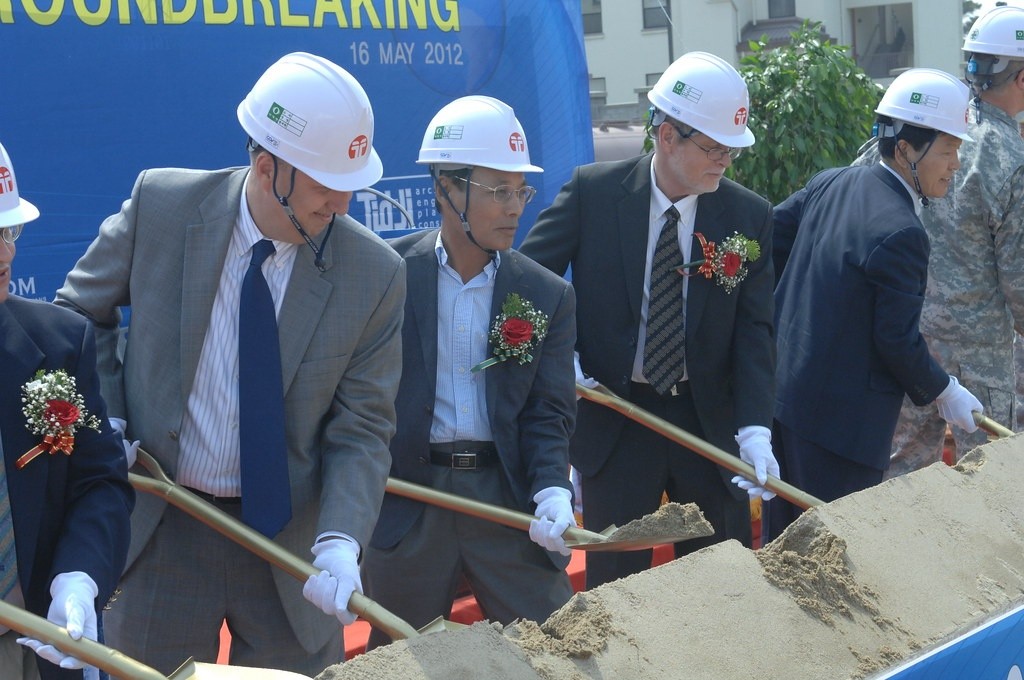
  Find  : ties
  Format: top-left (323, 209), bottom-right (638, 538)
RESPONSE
top-left (238, 239), bottom-right (293, 540)
top-left (642, 206), bottom-right (685, 399)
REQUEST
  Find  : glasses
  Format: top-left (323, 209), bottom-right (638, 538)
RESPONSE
top-left (455, 176), bottom-right (537, 203)
top-left (674, 126), bottom-right (743, 161)
top-left (0, 224), bottom-right (24, 244)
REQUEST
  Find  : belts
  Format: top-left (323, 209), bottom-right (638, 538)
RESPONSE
top-left (429, 448), bottom-right (499, 470)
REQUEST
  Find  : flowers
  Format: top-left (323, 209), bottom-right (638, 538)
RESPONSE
top-left (22, 366), bottom-right (107, 438)
top-left (489, 291), bottom-right (550, 356)
top-left (712, 229), bottom-right (760, 294)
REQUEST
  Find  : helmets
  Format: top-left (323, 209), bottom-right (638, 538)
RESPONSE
top-left (0, 141), bottom-right (40, 228)
top-left (873, 67), bottom-right (975, 143)
top-left (647, 50), bottom-right (755, 147)
top-left (961, 6), bottom-right (1024, 57)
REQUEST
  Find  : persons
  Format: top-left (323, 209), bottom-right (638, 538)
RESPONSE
top-left (51, 50), bottom-right (408, 680)
top-left (359, 95), bottom-right (576, 658)
top-left (851, 5), bottom-right (1024, 481)
top-left (766, 69), bottom-right (981, 549)
top-left (0, 144), bottom-right (137, 680)
top-left (516, 50), bottom-right (781, 598)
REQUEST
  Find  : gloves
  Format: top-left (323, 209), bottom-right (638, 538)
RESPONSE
top-left (16, 572), bottom-right (99, 669)
top-left (528, 487), bottom-right (578, 556)
top-left (936, 375), bottom-right (983, 433)
top-left (732, 426), bottom-right (780, 501)
top-left (108, 417), bottom-right (140, 470)
top-left (303, 539), bottom-right (364, 625)
top-left (573, 349), bottom-right (600, 401)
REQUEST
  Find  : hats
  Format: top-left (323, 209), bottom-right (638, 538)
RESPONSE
top-left (415, 95), bottom-right (544, 174)
top-left (237, 52), bottom-right (384, 192)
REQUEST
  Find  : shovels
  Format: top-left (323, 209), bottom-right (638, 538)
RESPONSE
top-left (121, 443), bottom-right (449, 643)
top-left (0, 600), bottom-right (318, 680)
top-left (386, 479), bottom-right (716, 552)
